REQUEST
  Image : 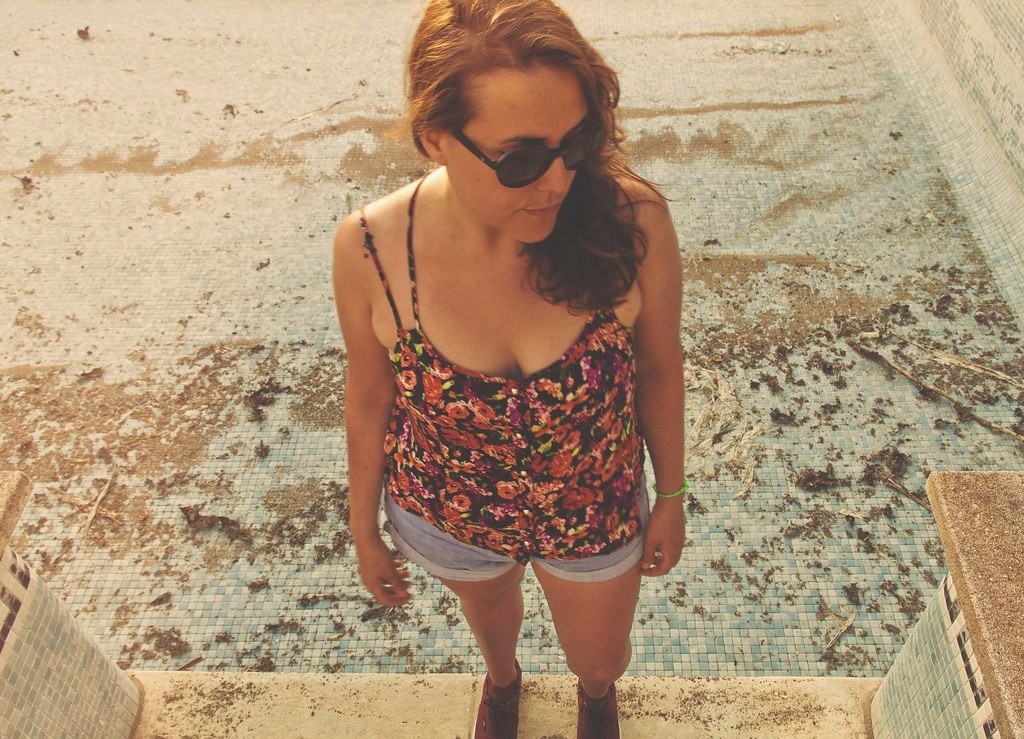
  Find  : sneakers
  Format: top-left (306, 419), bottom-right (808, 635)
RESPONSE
top-left (474, 660), bottom-right (522, 739)
top-left (576, 679), bottom-right (621, 739)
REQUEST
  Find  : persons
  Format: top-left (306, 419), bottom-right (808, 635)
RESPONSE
top-left (329, 0), bottom-right (687, 739)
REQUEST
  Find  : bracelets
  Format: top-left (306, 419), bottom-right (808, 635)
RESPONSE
top-left (650, 477), bottom-right (691, 498)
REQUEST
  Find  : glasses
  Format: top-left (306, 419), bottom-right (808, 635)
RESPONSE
top-left (441, 119), bottom-right (601, 187)
